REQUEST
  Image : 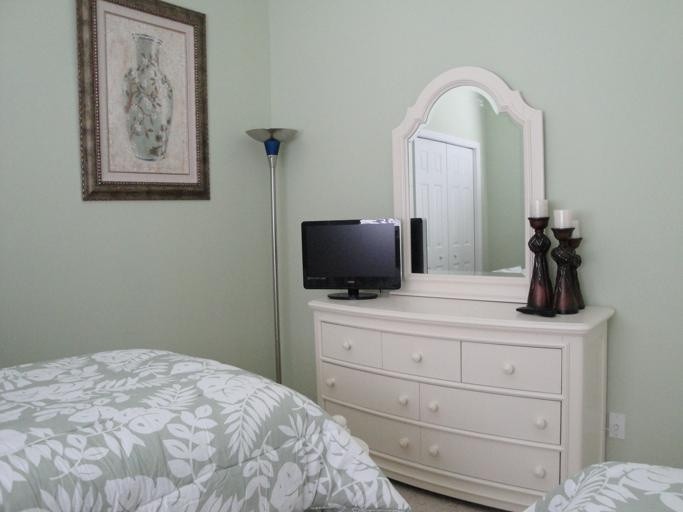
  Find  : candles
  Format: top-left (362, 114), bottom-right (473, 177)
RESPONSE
top-left (569, 220), bottom-right (580, 239)
top-left (528, 198), bottom-right (547, 218)
top-left (553, 210), bottom-right (571, 228)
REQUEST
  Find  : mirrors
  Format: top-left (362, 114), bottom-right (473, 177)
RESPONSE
top-left (388, 65), bottom-right (546, 304)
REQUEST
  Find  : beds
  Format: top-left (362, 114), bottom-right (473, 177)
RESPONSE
top-left (0, 346), bottom-right (410, 511)
top-left (522, 460), bottom-right (683, 512)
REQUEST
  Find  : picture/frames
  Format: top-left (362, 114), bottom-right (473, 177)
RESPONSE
top-left (77, 0), bottom-right (211, 203)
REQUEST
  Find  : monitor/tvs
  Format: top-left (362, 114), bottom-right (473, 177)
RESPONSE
top-left (411, 218), bottom-right (428, 274)
top-left (301, 218), bottom-right (402, 300)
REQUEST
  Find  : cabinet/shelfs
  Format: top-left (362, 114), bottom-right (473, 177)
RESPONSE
top-left (308, 292), bottom-right (614, 512)
